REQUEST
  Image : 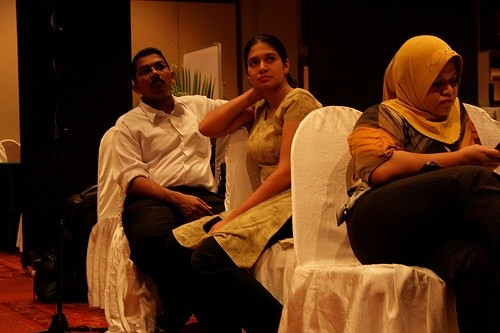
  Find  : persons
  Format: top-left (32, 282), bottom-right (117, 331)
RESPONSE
top-left (155, 31), bottom-right (324, 333)
top-left (336, 32), bottom-right (500, 333)
top-left (113, 47), bottom-right (253, 333)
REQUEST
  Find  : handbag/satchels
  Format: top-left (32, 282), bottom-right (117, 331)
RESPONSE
top-left (29, 235), bottom-right (58, 304)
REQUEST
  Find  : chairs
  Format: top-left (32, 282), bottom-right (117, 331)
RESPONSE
top-left (86, 106), bottom-right (500, 333)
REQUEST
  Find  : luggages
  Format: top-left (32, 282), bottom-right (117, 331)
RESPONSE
top-left (56, 184), bottom-right (102, 304)
